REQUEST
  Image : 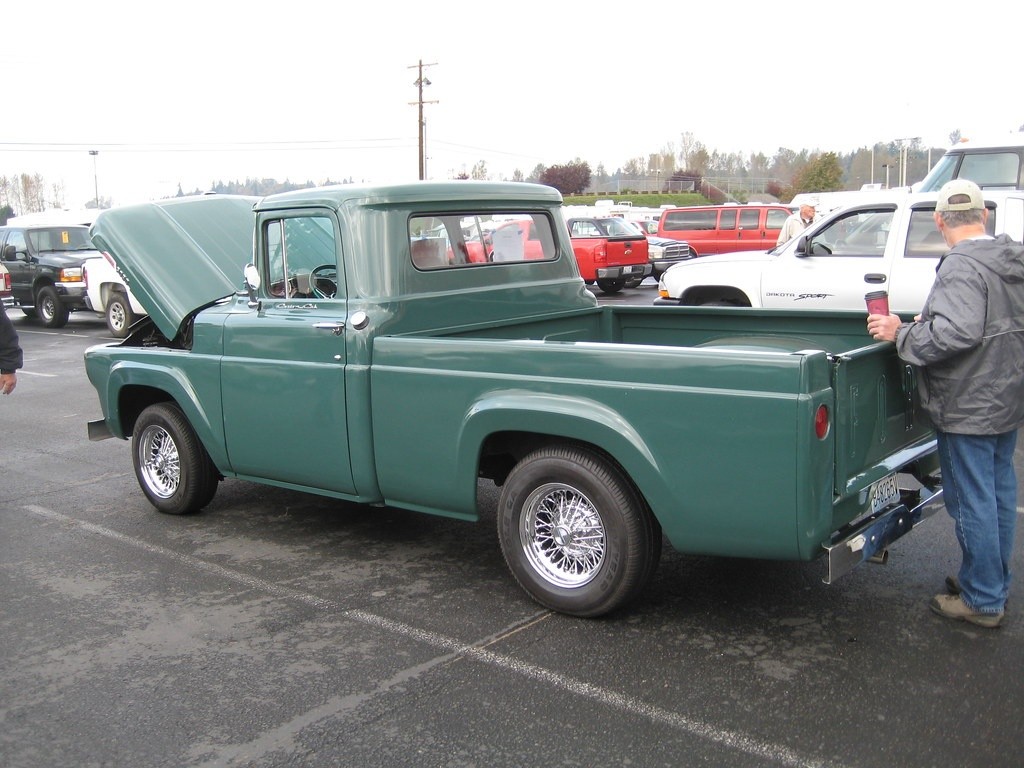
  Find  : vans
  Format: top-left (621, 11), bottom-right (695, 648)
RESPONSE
top-left (905, 137), bottom-right (1024, 242)
top-left (657, 203), bottom-right (799, 259)
top-left (789, 184), bottom-right (911, 216)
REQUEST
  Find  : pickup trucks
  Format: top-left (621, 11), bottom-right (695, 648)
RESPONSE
top-left (447, 217), bottom-right (653, 294)
top-left (84, 256), bottom-right (148, 337)
top-left (83, 181), bottom-right (947, 619)
top-left (651, 190), bottom-right (1024, 315)
top-left (566, 217), bottom-right (691, 289)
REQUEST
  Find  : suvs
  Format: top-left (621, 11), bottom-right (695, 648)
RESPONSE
top-left (0, 225), bottom-right (102, 329)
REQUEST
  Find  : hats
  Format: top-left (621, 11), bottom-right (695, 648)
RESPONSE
top-left (800, 195), bottom-right (820, 207)
top-left (936, 177), bottom-right (985, 212)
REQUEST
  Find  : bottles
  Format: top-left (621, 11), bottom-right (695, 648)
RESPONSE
top-left (864, 290), bottom-right (889, 316)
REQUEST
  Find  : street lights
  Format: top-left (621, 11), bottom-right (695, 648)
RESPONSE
top-left (88, 150), bottom-right (99, 201)
top-left (882, 163), bottom-right (895, 190)
top-left (865, 142), bottom-right (874, 183)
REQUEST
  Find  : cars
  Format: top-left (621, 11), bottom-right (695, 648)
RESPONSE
top-left (438, 200), bottom-right (675, 232)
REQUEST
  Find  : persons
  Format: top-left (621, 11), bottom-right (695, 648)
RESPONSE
top-left (776, 198), bottom-right (821, 246)
top-left (867, 180), bottom-right (1024, 627)
top-left (0, 297), bottom-right (23, 395)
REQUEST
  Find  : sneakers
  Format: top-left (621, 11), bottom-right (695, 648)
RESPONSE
top-left (928, 594), bottom-right (1004, 628)
top-left (945, 576), bottom-right (961, 593)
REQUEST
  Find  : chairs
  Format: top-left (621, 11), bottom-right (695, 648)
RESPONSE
top-left (411, 238), bottom-right (448, 268)
